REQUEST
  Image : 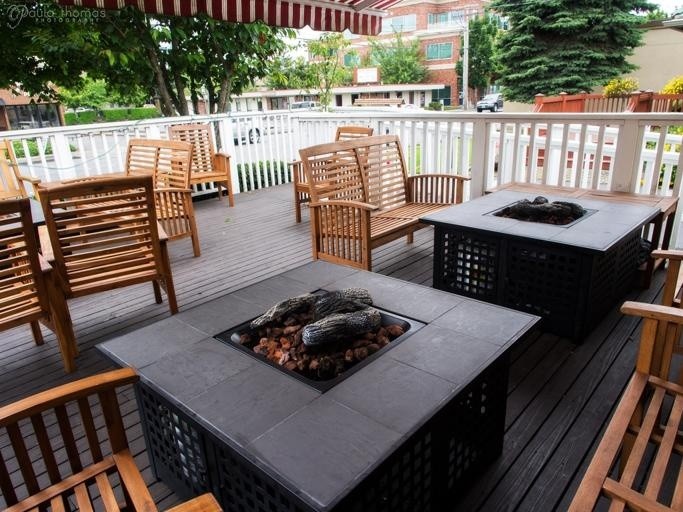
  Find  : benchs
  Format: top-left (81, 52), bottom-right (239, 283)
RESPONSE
top-left (565, 298), bottom-right (682, 512)
top-left (629, 247), bottom-right (682, 445)
top-left (298, 134), bottom-right (471, 272)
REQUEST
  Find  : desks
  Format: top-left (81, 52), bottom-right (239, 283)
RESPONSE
top-left (487, 181), bottom-right (679, 289)
top-left (97, 259), bottom-right (539, 512)
top-left (418, 190), bottom-right (662, 351)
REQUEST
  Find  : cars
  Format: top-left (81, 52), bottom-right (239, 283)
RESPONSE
top-left (217, 111), bottom-right (276, 144)
top-left (291, 101), bottom-right (326, 112)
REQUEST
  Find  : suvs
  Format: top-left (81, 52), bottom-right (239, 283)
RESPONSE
top-left (476, 93), bottom-right (503, 112)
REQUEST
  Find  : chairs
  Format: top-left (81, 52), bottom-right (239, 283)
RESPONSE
top-left (0, 369), bottom-right (223, 512)
top-left (291, 126), bottom-right (371, 223)
